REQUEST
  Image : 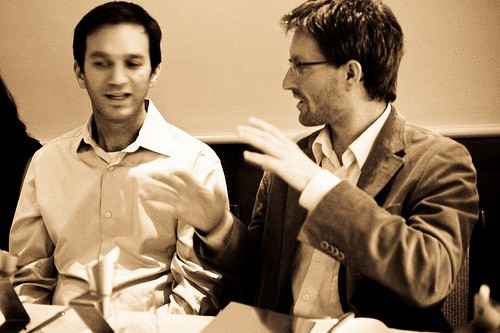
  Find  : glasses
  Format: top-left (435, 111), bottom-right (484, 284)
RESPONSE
top-left (288, 57), bottom-right (368, 73)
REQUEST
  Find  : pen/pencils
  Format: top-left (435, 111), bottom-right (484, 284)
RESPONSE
top-left (328, 311), bottom-right (355, 333)
top-left (28, 311), bottom-right (65, 333)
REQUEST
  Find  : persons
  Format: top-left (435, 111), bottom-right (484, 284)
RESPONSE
top-left (142, 1), bottom-right (481, 333)
top-left (0, 0), bottom-right (230, 317)
top-left (0, 76), bottom-right (43, 250)
